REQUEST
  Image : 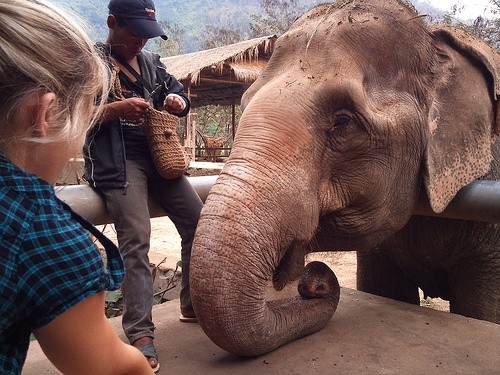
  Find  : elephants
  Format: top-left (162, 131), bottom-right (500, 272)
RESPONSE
top-left (188, 0), bottom-right (500, 359)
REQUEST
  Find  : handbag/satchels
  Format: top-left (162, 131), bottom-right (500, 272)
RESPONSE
top-left (142, 106), bottom-right (191, 178)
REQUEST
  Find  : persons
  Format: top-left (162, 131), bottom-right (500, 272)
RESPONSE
top-left (83, 0), bottom-right (204, 372)
top-left (0, 0), bottom-right (156, 375)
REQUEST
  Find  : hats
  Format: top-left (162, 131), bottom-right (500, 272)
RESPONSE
top-left (108, 0), bottom-right (167, 40)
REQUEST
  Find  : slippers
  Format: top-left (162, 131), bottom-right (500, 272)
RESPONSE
top-left (136, 345), bottom-right (160, 372)
top-left (180, 314), bottom-right (198, 323)
